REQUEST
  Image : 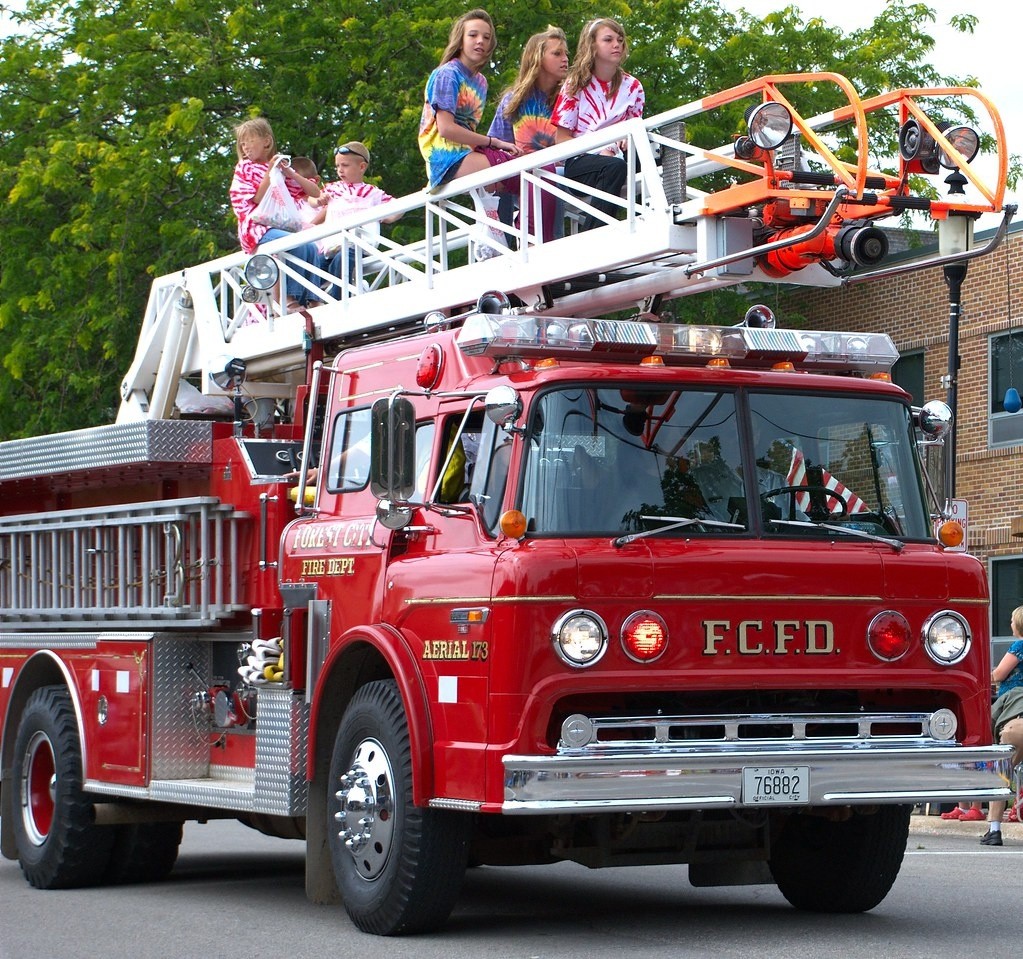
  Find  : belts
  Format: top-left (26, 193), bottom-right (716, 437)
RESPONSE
top-left (1000, 713), bottom-right (1023, 727)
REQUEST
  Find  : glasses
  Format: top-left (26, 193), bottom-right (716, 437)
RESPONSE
top-left (333, 147), bottom-right (368, 164)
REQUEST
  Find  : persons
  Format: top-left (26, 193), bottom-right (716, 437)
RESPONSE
top-left (550, 18), bottom-right (662, 231)
top-left (488, 28), bottom-right (569, 239)
top-left (459, 443), bottom-right (570, 533)
top-left (285, 157), bottom-right (327, 255)
top-left (980, 687), bottom-right (1023, 845)
top-left (681, 413), bottom-right (810, 524)
top-left (941, 800), bottom-right (986, 821)
top-left (418, 9), bottom-right (555, 252)
top-left (308, 142), bottom-right (406, 300)
top-left (228, 119), bottom-right (325, 315)
top-left (990, 606), bottom-right (1023, 696)
top-left (284, 422), bottom-right (467, 504)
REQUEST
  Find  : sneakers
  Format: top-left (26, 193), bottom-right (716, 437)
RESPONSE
top-left (980, 829), bottom-right (1002, 845)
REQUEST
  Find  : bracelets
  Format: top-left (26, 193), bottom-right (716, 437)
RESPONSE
top-left (487, 136), bottom-right (491, 147)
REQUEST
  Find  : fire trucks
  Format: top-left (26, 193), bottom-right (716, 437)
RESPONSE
top-left (0, 70), bottom-right (1017, 936)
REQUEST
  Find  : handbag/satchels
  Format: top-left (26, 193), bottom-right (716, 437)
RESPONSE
top-left (470, 185), bottom-right (509, 262)
top-left (249, 154), bottom-right (303, 232)
top-left (321, 189), bottom-right (381, 260)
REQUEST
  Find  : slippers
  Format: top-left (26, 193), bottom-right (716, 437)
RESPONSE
top-left (940, 807), bottom-right (965, 819)
top-left (959, 807), bottom-right (986, 820)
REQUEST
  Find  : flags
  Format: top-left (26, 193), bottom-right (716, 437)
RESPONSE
top-left (786, 448), bottom-right (866, 513)
